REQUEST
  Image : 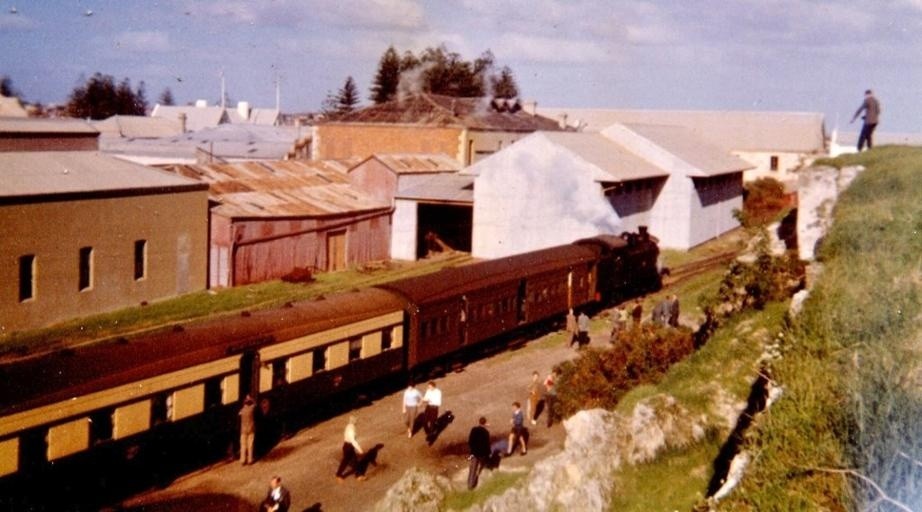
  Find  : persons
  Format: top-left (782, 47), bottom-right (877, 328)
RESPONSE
top-left (849, 89), bottom-right (882, 151)
top-left (564, 295), bottom-right (681, 348)
top-left (467, 416), bottom-right (493, 488)
top-left (260, 473), bottom-right (292, 511)
top-left (503, 402), bottom-right (527, 457)
top-left (402, 378), bottom-right (423, 438)
top-left (527, 368), bottom-right (546, 425)
top-left (422, 381), bottom-right (442, 439)
top-left (235, 393), bottom-right (257, 465)
top-left (334, 414), bottom-right (368, 483)
top-left (544, 362), bottom-right (563, 429)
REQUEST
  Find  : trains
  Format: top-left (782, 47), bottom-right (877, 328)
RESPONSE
top-left (0, 226), bottom-right (659, 507)
